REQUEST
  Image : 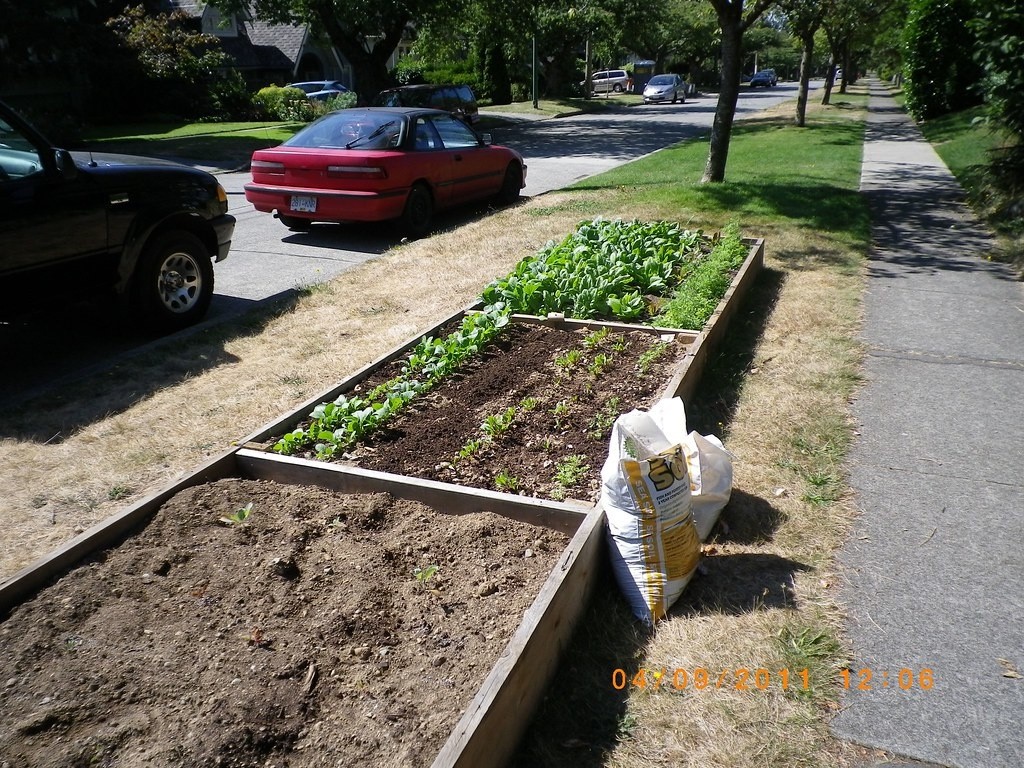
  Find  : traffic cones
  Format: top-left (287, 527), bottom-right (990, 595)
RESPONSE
top-left (623, 79), bottom-right (632, 94)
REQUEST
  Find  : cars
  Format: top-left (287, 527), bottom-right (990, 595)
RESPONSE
top-left (572, 69), bottom-right (630, 93)
top-left (0, 100), bottom-right (236, 340)
top-left (642, 73), bottom-right (687, 105)
top-left (835, 68), bottom-right (842, 80)
top-left (241, 105), bottom-right (528, 241)
top-left (750, 68), bottom-right (777, 88)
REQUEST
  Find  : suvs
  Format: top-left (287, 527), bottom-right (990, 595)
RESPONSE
top-left (365, 81), bottom-right (481, 126)
top-left (282, 80), bottom-right (351, 102)
